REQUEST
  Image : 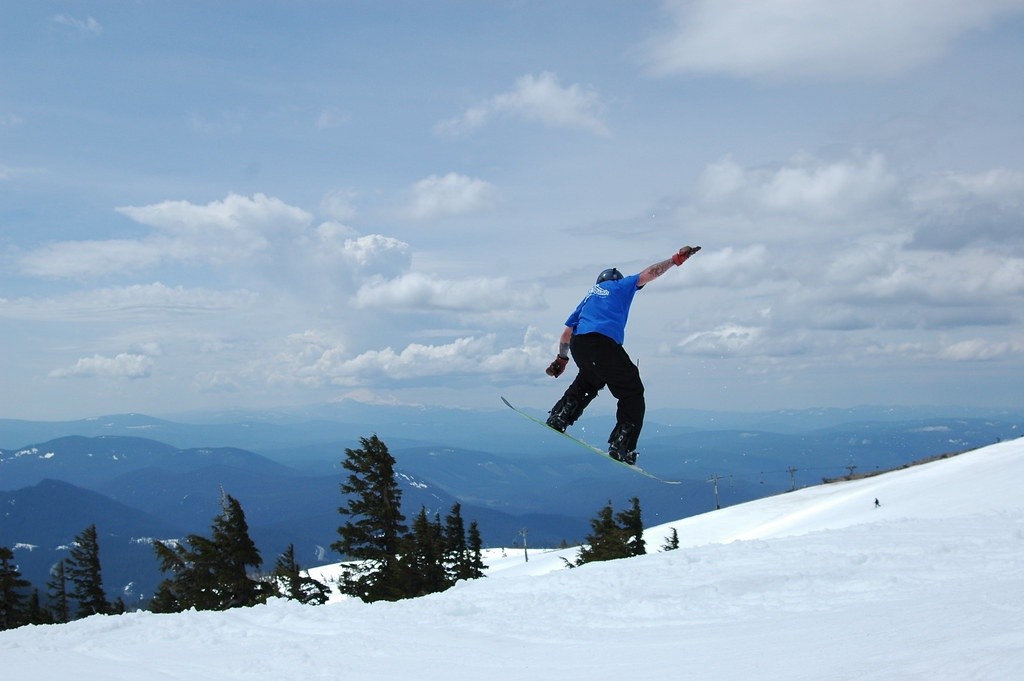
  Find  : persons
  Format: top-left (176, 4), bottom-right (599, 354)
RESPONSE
top-left (544, 245), bottom-right (703, 465)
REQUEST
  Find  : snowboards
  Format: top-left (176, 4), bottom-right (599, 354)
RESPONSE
top-left (501, 396), bottom-right (684, 484)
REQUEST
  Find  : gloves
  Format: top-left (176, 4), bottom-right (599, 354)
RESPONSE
top-left (545, 354), bottom-right (570, 379)
top-left (672, 246), bottom-right (702, 266)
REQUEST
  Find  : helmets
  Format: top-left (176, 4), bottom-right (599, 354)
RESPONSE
top-left (596, 267), bottom-right (623, 283)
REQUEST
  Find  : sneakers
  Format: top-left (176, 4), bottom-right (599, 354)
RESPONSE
top-left (608, 443), bottom-right (637, 465)
top-left (547, 414), bottom-right (567, 433)
